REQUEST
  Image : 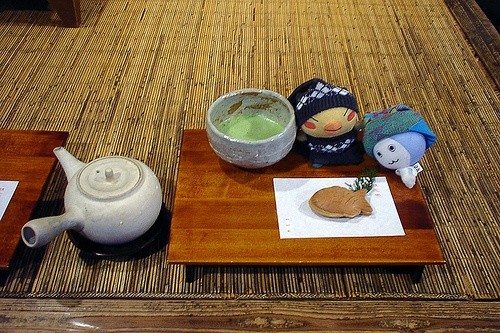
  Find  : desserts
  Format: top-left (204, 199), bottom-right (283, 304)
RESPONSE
top-left (309, 185), bottom-right (373, 219)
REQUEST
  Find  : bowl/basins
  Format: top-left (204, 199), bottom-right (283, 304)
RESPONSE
top-left (204, 87), bottom-right (298, 168)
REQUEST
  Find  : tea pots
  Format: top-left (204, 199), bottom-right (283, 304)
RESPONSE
top-left (20, 146), bottom-right (163, 249)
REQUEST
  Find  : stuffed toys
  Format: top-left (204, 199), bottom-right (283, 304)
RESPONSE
top-left (288, 78), bottom-right (365, 169)
top-left (358, 104), bottom-right (438, 189)
